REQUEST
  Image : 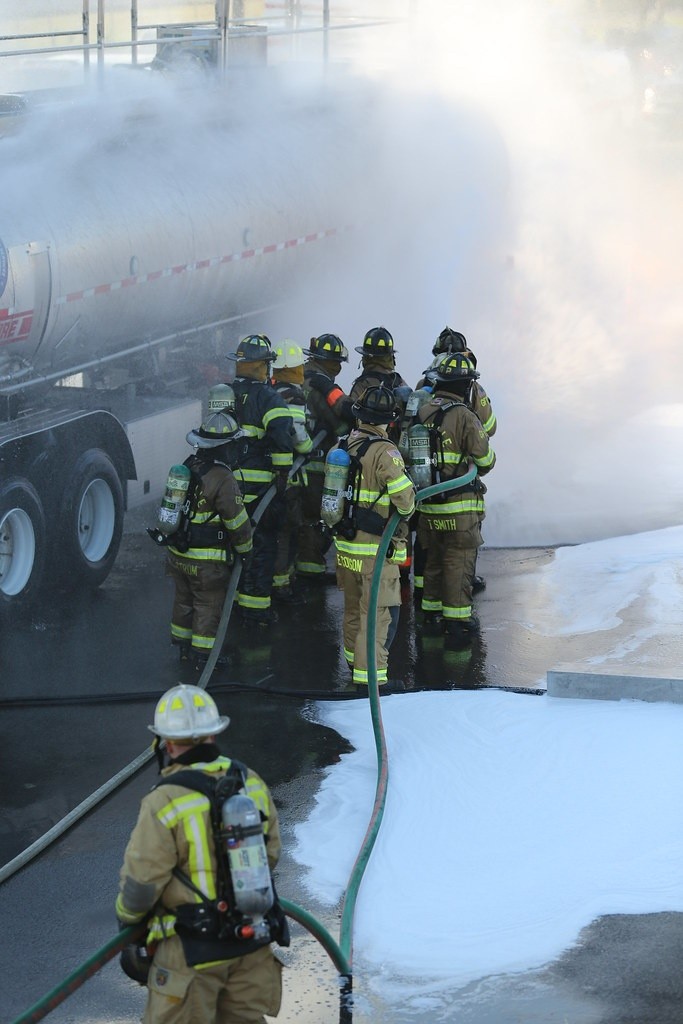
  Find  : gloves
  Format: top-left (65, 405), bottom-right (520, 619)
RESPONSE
top-left (238, 550), bottom-right (253, 573)
top-left (272, 471), bottom-right (287, 494)
top-left (116, 926), bottom-right (153, 985)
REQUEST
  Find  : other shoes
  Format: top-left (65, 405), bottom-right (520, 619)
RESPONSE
top-left (471, 575), bottom-right (486, 589)
top-left (322, 571), bottom-right (336, 586)
top-left (423, 612), bottom-right (444, 623)
top-left (270, 585), bottom-right (304, 605)
top-left (241, 608), bottom-right (281, 625)
top-left (194, 653), bottom-right (234, 671)
top-left (356, 680), bottom-right (405, 692)
top-left (445, 617), bottom-right (479, 633)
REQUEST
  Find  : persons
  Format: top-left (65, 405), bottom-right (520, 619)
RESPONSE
top-left (326, 382), bottom-right (415, 694)
top-left (416, 325), bottom-right (497, 594)
top-left (223, 335), bottom-right (297, 632)
top-left (271, 339), bottom-right (314, 611)
top-left (168, 411), bottom-right (254, 676)
top-left (297, 332), bottom-right (349, 586)
top-left (349, 326), bottom-right (413, 585)
top-left (114, 684), bottom-right (290, 1024)
top-left (416, 353), bottom-right (496, 639)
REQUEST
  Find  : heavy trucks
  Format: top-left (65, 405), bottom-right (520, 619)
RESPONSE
top-left (0, 0), bottom-right (527, 605)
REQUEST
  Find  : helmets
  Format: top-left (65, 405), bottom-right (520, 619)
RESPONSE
top-left (432, 326), bottom-right (470, 356)
top-left (351, 387), bottom-right (398, 424)
top-left (270, 340), bottom-right (309, 369)
top-left (424, 353), bottom-right (475, 381)
top-left (185, 412), bottom-right (244, 450)
top-left (354, 327), bottom-right (395, 358)
top-left (225, 334), bottom-right (275, 361)
top-left (300, 334), bottom-right (348, 361)
top-left (145, 684), bottom-right (230, 740)
